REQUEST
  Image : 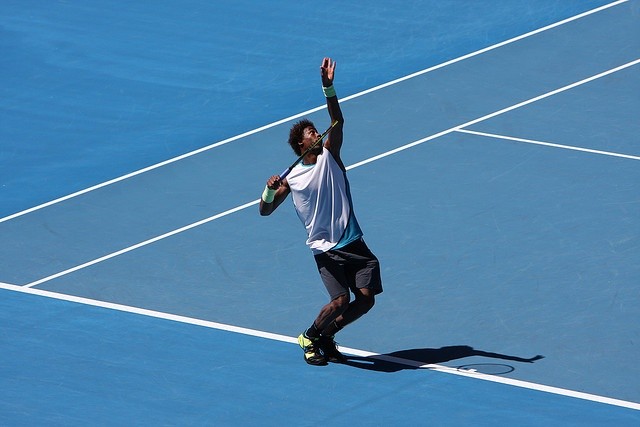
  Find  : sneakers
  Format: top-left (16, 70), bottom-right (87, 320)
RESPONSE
top-left (321, 333), bottom-right (347, 361)
top-left (298, 328), bottom-right (325, 365)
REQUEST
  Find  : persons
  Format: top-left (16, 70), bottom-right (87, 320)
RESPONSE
top-left (258, 56), bottom-right (383, 366)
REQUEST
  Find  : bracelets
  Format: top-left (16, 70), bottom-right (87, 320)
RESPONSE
top-left (321, 84), bottom-right (336, 97)
top-left (261, 184), bottom-right (277, 203)
